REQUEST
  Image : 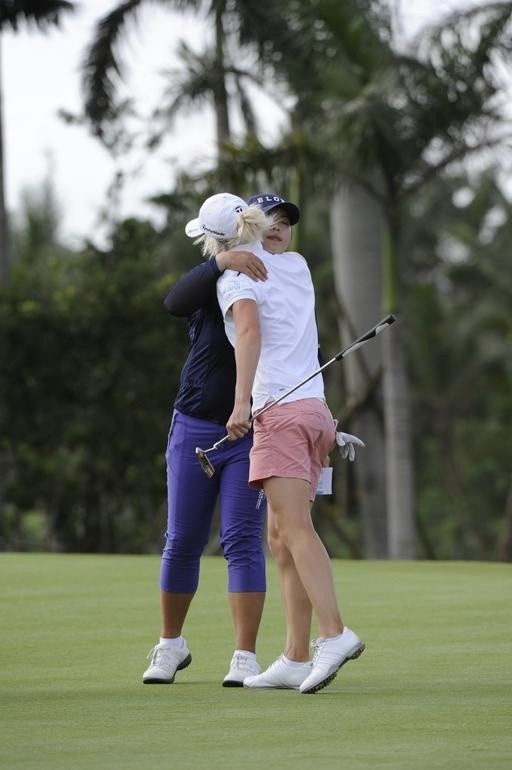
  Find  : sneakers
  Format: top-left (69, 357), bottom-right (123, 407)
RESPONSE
top-left (222, 626), bottom-right (366, 694)
top-left (143, 638), bottom-right (192, 684)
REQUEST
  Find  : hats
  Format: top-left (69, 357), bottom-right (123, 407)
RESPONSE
top-left (185, 193), bottom-right (300, 238)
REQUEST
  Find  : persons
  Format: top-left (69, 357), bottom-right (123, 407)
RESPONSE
top-left (144, 193), bottom-right (300, 688)
top-left (185, 194), bottom-right (365, 694)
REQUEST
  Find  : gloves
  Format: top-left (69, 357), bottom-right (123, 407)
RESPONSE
top-left (335, 431), bottom-right (366, 462)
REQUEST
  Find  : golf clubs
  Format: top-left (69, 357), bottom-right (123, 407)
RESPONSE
top-left (196, 315), bottom-right (395, 479)
